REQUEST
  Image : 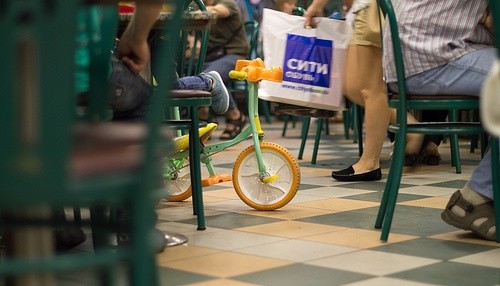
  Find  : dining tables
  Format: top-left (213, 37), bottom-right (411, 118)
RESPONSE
top-left (73, 10), bottom-right (210, 251)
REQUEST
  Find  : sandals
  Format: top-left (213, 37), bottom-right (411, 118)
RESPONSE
top-left (219, 111), bottom-right (248, 140)
top-left (199, 118), bottom-right (219, 126)
top-left (441, 190), bottom-right (496, 241)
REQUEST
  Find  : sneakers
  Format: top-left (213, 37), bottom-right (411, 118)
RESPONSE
top-left (205, 71), bottom-right (229, 114)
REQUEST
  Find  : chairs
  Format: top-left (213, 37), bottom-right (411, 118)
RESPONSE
top-left (227, 9), bottom-right (462, 175)
top-left (1, 1), bottom-right (210, 285)
top-left (140, 89), bottom-right (211, 230)
top-left (375, 0), bottom-right (490, 243)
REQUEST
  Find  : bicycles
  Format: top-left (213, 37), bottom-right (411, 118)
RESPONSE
top-left (163, 58), bottom-right (302, 211)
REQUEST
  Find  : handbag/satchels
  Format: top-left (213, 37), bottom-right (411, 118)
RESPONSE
top-left (258, 8), bottom-right (350, 112)
top-left (108, 60), bottom-right (151, 112)
top-left (205, 45), bottom-right (226, 63)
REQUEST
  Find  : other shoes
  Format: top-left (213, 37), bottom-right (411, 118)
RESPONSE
top-left (53, 226), bottom-right (87, 253)
top-left (117, 228), bottom-right (166, 257)
top-left (422, 151), bottom-right (439, 165)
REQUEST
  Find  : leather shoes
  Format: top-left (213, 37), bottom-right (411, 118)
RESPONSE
top-left (332, 165), bottom-right (382, 182)
top-left (404, 154), bottom-right (421, 166)
